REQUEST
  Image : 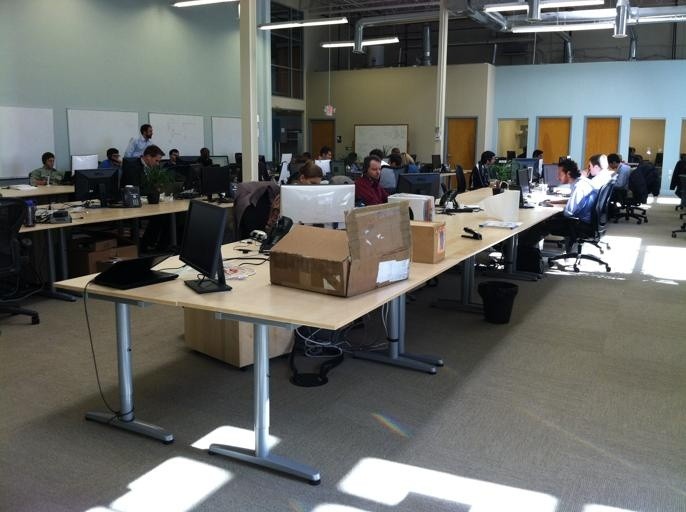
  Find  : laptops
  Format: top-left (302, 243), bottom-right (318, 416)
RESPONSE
top-left (92, 252), bottom-right (179, 290)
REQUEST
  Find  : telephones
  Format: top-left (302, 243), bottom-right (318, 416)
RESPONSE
top-left (228, 182), bottom-right (241, 198)
top-left (120, 186), bottom-right (142, 208)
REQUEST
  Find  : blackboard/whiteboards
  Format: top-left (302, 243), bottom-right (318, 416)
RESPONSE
top-left (211, 116), bottom-right (242, 157)
top-left (66, 108), bottom-right (139, 163)
top-left (354, 123), bottom-right (408, 163)
top-left (148, 112), bottom-right (205, 161)
top-left (0, 106), bottom-right (56, 180)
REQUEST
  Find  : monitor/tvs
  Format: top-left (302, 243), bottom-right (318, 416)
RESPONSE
top-left (654, 153), bottom-right (663, 167)
top-left (179, 199), bottom-right (232, 294)
top-left (74, 169), bottom-right (119, 208)
top-left (71, 154), bottom-right (99, 176)
top-left (396, 173), bottom-right (440, 207)
top-left (176, 156), bottom-right (201, 181)
top-left (329, 160), bottom-right (347, 176)
top-left (122, 157), bottom-right (139, 169)
top-left (280, 184), bottom-right (356, 232)
top-left (506, 150), bottom-right (572, 208)
top-left (432, 155), bottom-right (441, 169)
top-left (315, 159), bottom-right (332, 177)
top-left (210, 155), bottom-right (229, 169)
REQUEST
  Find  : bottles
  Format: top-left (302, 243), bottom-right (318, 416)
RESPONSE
top-left (25, 201), bottom-right (36, 226)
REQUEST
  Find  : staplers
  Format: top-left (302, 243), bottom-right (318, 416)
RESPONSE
top-left (461, 227), bottom-right (482, 240)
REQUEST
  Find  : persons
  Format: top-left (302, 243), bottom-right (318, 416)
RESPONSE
top-left (113, 145), bottom-right (165, 196)
top-left (236, 161), bottom-right (323, 243)
top-left (122, 124), bottom-right (154, 160)
top-left (517, 146), bottom-right (527, 158)
top-left (313, 146), bottom-right (332, 160)
top-left (29, 152), bottom-right (63, 205)
top-left (164, 148), bottom-right (179, 168)
top-left (468, 151), bottom-right (496, 191)
top-left (517, 159), bottom-right (597, 249)
top-left (99, 148), bottom-right (122, 178)
top-left (588, 153), bottom-right (641, 195)
top-left (532, 149), bottom-right (543, 158)
top-left (195, 148), bottom-right (212, 166)
top-left (355, 147), bottom-right (419, 206)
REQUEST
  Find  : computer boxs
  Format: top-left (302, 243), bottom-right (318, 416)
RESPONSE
top-left (387, 193), bottom-right (435, 220)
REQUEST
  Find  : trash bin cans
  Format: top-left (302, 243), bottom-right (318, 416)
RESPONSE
top-left (478, 281), bottom-right (519, 325)
